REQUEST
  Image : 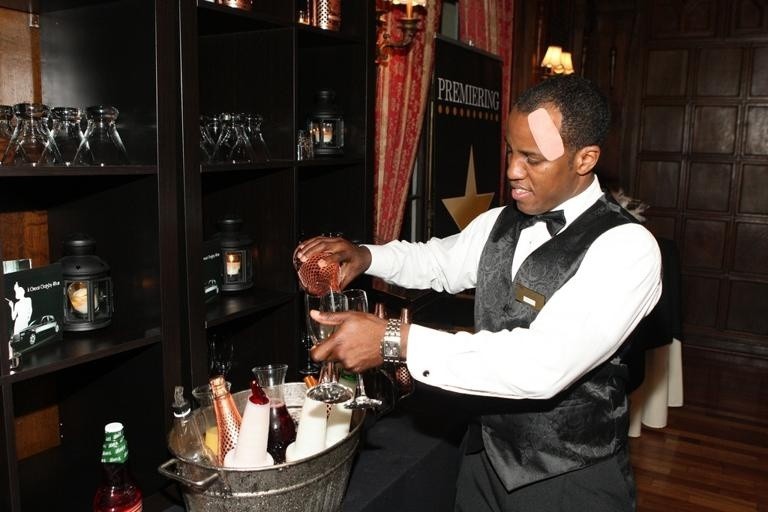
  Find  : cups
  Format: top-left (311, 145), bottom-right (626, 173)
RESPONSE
top-left (199, 112), bottom-right (273, 162)
top-left (207, 332), bottom-right (236, 383)
top-left (0, 103), bottom-right (134, 166)
top-left (296, 128), bottom-right (314, 162)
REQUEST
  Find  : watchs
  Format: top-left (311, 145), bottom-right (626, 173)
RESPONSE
top-left (379, 317), bottom-right (402, 366)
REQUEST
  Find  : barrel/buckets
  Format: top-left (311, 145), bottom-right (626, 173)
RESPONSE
top-left (158, 382), bottom-right (366, 512)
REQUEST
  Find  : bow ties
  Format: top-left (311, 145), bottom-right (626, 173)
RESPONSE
top-left (519, 209), bottom-right (566, 237)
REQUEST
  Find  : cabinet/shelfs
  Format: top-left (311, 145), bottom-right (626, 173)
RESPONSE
top-left (0, 1), bottom-right (187, 511)
top-left (177, 0), bottom-right (374, 406)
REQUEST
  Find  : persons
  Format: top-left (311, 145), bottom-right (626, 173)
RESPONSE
top-left (295, 72), bottom-right (667, 511)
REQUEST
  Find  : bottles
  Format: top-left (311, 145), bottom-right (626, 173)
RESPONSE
top-left (171, 363), bottom-right (359, 469)
top-left (90, 421), bottom-right (143, 512)
top-left (292, 244), bottom-right (344, 298)
top-left (362, 365), bottom-right (398, 417)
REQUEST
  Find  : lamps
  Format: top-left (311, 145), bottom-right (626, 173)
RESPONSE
top-left (541, 46), bottom-right (575, 82)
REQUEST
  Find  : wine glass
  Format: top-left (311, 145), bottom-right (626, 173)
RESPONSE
top-left (304, 289), bottom-right (383, 409)
